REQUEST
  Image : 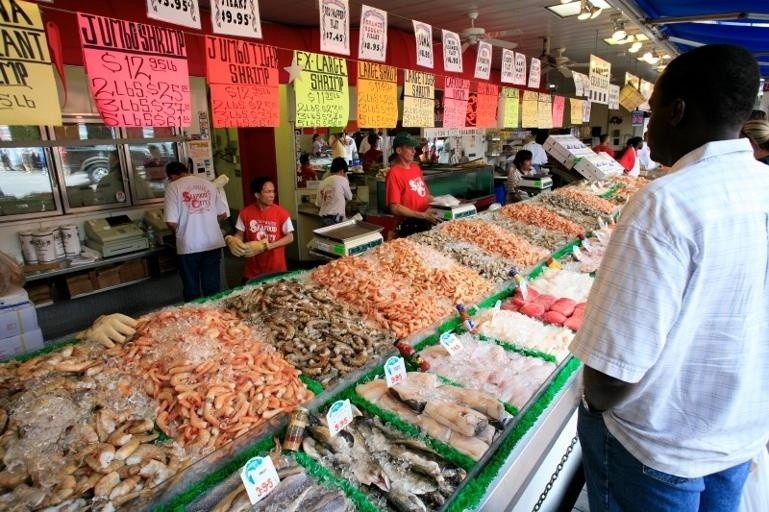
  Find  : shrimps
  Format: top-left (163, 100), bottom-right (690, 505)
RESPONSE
top-left (536, 186), bottom-right (616, 225)
top-left (0, 340), bottom-right (159, 512)
top-left (381, 231), bottom-right (517, 299)
top-left (451, 207), bottom-right (572, 264)
top-left (122, 306), bottom-right (314, 450)
top-left (314, 253), bottom-right (450, 339)
top-left (227, 279), bottom-right (390, 386)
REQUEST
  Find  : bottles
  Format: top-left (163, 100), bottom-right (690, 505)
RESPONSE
top-left (146, 225), bottom-right (155, 248)
top-left (456, 302), bottom-right (475, 332)
top-left (508, 268), bottom-right (524, 286)
top-left (392, 338), bottom-right (431, 373)
top-left (283, 407), bottom-right (310, 450)
top-left (544, 255), bottom-right (561, 269)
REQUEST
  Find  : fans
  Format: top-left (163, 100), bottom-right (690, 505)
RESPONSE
top-left (450, 12), bottom-right (523, 56)
top-left (536, 47), bottom-right (591, 79)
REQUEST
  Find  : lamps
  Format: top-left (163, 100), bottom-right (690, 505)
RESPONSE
top-left (587, 0), bottom-right (602, 20)
top-left (577, 0), bottom-right (591, 21)
top-left (610, 15), bottom-right (659, 64)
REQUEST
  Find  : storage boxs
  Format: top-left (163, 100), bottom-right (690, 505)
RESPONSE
top-left (0, 327), bottom-right (46, 361)
top-left (542, 134), bottom-right (596, 171)
top-left (573, 151), bottom-right (626, 181)
top-left (0, 287), bottom-right (39, 340)
top-left (65, 257), bottom-right (148, 297)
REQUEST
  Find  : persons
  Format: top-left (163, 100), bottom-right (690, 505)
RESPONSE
top-left (300, 127), bottom-right (442, 237)
top-left (568, 43), bottom-right (769, 512)
top-left (0, 149), bottom-right (37, 175)
top-left (224, 176), bottom-right (295, 289)
top-left (739, 120), bottom-right (769, 165)
top-left (492, 130), bottom-right (549, 206)
top-left (162, 161), bottom-right (228, 304)
top-left (590, 133), bottom-right (662, 179)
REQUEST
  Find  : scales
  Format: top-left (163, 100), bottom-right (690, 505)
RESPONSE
top-left (425, 199), bottom-right (478, 221)
top-left (516, 174), bottom-right (553, 190)
top-left (306, 217), bottom-right (385, 259)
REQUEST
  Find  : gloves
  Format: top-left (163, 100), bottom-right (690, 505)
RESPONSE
top-left (243, 237), bottom-right (271, 258)
top-left (88, 312), bottom-right (139, 349)
top-left (224, 235), bottom-right (249, 258)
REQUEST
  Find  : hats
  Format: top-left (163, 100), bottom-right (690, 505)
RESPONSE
top-left (392, 129), bottom-right (424, 149)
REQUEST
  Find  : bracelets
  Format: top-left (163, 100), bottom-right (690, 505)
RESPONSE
top-left (581, 391), bottom-right (608, 414)
